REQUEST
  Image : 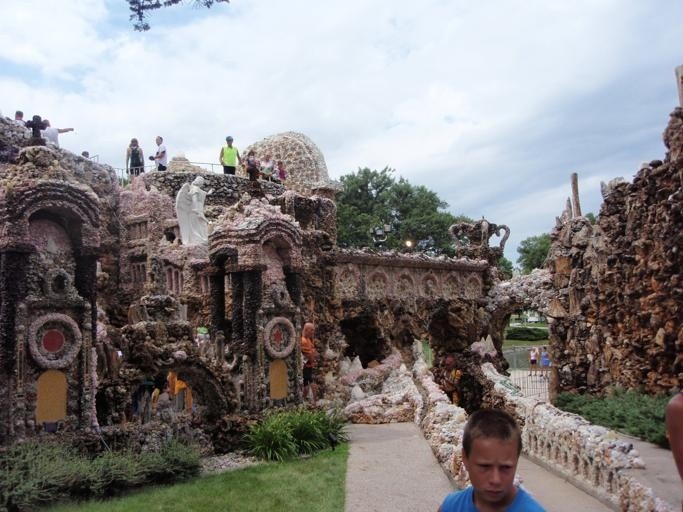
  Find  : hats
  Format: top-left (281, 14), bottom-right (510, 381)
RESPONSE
top-left (226, 136), bottom-right (232, 140)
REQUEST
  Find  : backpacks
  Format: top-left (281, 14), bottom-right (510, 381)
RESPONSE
top-left (130, 147), bottom-right (141, 167)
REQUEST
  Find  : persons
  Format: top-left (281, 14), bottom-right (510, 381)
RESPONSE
top-left (666, 388), bottom-right (683, 479)
top-left (125, 138), bottom-right (145, 183)
top-left (42, 119), bottom-right (74, 147)
top-left (219, 136), bottom-right (245, 175)
top-left (176, 175), bottom-right (215, 264)
top-left (82, 151), bottom-right (90, 159)
top-left (14, 110), bottom-right (26, 125)
top-left (150, 136), bottom-right (167, 171)
top-left (300, 322), bottom-right (318, 401)
top-left (528, 347), bottom-right (539, 376)
top-left (241, 150), bottom-right (289, 185)
top-left (438, 408), bottom-right (547, 512)
top-left (540, 347), bottom-right (552, 377)
